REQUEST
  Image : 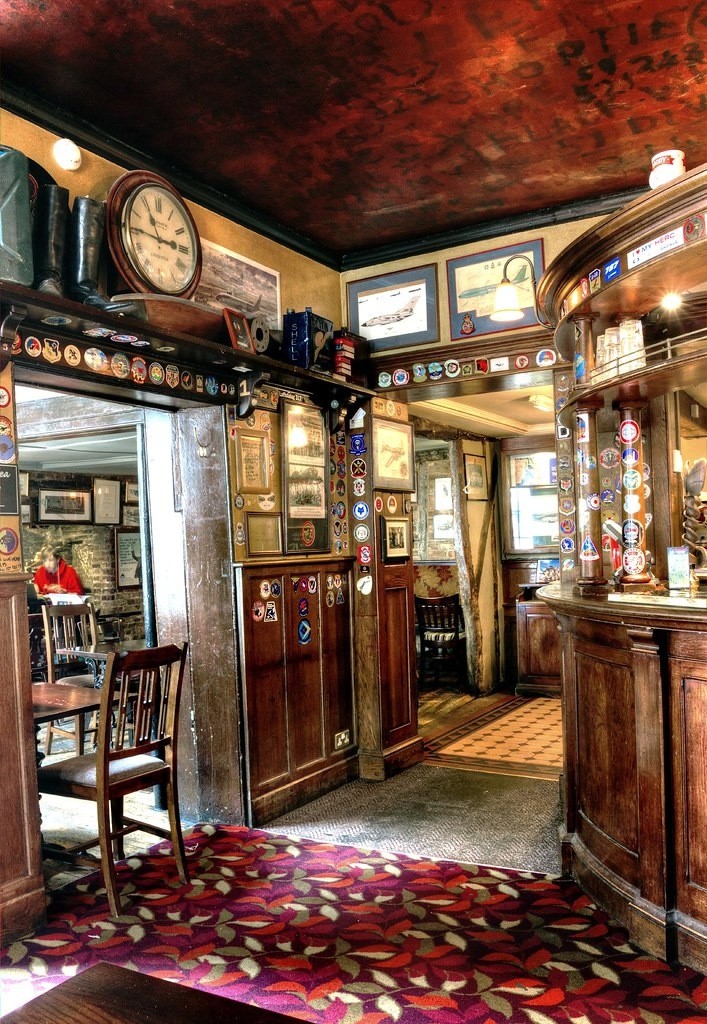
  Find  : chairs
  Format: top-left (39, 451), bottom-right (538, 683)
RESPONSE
top-left (414, 594), bottom-right (466, 693)
top-left (28, 603), bottom-right (189, 918)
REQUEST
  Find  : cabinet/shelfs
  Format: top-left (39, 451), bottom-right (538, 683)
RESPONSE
top-left (515, 593), bottom-right (565, 700)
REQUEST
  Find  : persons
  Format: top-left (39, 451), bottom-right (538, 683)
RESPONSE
top-left (33, 547), bottom-right (85, 595)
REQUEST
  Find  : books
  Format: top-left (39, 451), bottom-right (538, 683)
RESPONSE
top-left (332, 338), bottom-right (354, 381)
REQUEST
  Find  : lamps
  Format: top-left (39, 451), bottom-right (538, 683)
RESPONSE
top-left (490, 254), bottom-right (557, 329)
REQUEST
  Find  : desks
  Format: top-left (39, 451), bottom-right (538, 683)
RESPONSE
top-left (56, 640), bottom-right (147, 661)
top-left (32, 681), bottom-right (139, 858)
top-left (0, 961), bottom-right (323, 1024)
top-left (99, 611), bottom-right (143, 638)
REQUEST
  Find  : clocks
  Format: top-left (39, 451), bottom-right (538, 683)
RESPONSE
top-left (105, 170), bottom-right (203, 299)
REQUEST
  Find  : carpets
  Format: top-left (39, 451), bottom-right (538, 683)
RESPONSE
top-left (0, 822), bottom-right (707, 1024)
top-left (417, 695), bottom-right (563, 781)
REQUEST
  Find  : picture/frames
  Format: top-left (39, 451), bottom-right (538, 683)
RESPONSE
top-left (125, 481), bottom-right (138, 504)
top-left (242, 511), bottom-right (286, 558)
top-left (464, 453), bottom-right (488, 501)
top-left (278, 396), bottom-right (332, 558)
top-left (371, 413), bottom-right (416, 494)
top-left (222, 308), bottom-right (256, 354)
top-left (535, 559), bottom-right (560, 584)
top-left (115, 527), bottom-right (142, 590)
top-left (190, 236), bottom-right (282, 330)
top-left (445, 237), bottom-right (550, 342)
top-left (380, 515), bottom-right (411, 563)
top-left (345, 262), bottom-right (440, 353)
top-left (37, 488), bottom-right (93, 526)
